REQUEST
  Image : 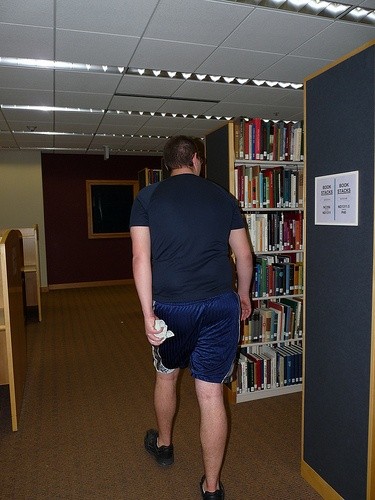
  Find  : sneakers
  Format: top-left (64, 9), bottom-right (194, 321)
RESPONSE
top-left (200, 474), bottom-right (225, 500)
top-left (143, 429), bottom-right (175, 466)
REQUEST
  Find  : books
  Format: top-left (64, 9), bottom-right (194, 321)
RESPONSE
top-left (231, 115), bottom-right (303, 394)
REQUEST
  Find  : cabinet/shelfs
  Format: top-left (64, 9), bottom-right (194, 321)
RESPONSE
top-left (300, 42), bottom-right (375, 500)
top-left (201, 118), bottom-right (303, 405)
top-left (0, 229), bottom-right (26, 433)
top-left (138, 168), bottom-right (164, 191)
top-left (16, 223), bottom-right (42, 322)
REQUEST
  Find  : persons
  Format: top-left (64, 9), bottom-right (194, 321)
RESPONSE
top-left (129, 135), bottom-right (253, 500)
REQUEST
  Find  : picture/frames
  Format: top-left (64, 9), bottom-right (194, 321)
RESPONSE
top-left (85, 179), bottom-right (140, 239)
